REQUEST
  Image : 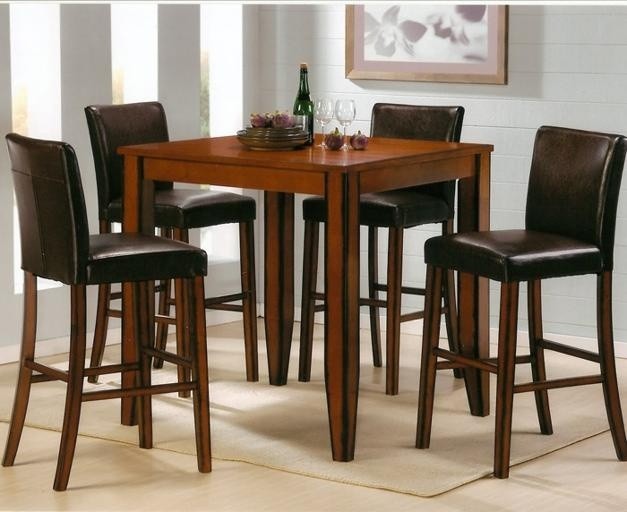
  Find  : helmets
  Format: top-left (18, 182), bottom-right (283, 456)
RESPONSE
top-left (235, 128), bottom-right (310, 151)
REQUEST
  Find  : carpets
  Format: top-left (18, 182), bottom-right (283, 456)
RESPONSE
top-left (0, 302), bottom-right (624, 502)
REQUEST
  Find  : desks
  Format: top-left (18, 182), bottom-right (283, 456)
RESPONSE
top-left (117, 133), bottom-right (494, 462)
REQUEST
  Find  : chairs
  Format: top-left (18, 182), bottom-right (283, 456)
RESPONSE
top-left (298, 104), bottom-right (464, 396)
top-left (1, 133), bottom-right (212, 486)
top-left (415, 126), bottom-right (626, 479)
top-left (85, 102), bottom-right (259, 398)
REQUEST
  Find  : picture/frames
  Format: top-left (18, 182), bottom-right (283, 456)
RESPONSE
top-left (343, 3), bottom-right (509, 87)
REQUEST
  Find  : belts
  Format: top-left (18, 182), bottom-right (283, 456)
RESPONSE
top-left (335, 99), bottom-right (357, 151)
top-left (315, 96), bottom-right (337, 149)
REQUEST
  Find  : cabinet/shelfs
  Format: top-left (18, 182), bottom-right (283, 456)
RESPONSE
top-left (292, 64), bottom-right (315, 146)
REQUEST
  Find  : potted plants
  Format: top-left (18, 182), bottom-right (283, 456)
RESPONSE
top-left (343, 3), bottom-right (509, 87)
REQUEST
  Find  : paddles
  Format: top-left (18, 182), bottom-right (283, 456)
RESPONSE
top-left (0, 302), bottom-right (624, 502)
